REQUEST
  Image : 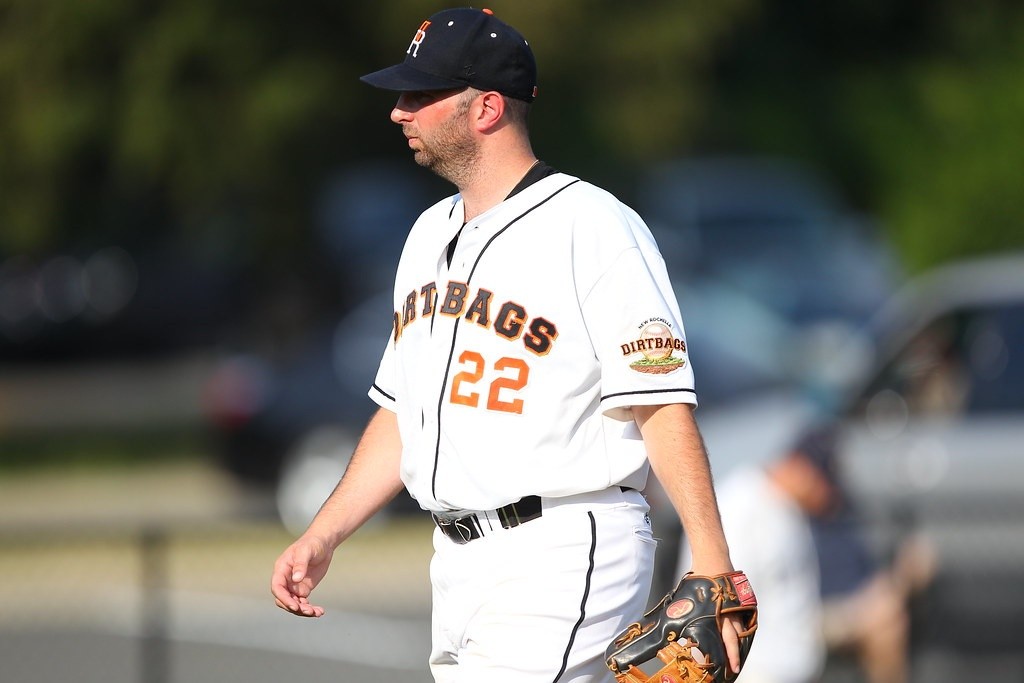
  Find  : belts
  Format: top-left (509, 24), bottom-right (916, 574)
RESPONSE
top-left (432, 480), bottom-right (634, 548)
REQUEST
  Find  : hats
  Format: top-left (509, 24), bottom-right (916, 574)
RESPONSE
top-left (357, 8), bottom-right (539, 105)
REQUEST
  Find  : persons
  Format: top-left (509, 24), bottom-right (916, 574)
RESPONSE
top-left (671, 430), bottom-right (914, 683)
top-left (272, 8), bottom-right (752, 683)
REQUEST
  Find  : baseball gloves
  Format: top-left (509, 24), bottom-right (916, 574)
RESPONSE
top-left (605, 569), bottom-right (758, 683)
